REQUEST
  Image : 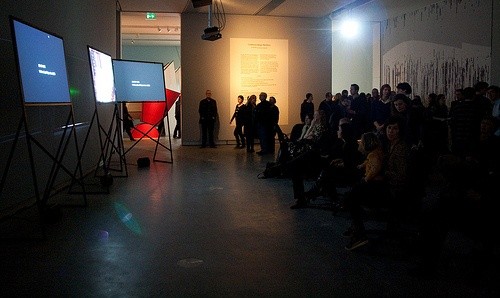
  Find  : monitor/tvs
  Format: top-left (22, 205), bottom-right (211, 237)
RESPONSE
top-left (112, 59), bottom-right (166, 103)
top-left (88, 45), bottom-right (117, 104)
top-left (10, 15), bottom-right (73, 107)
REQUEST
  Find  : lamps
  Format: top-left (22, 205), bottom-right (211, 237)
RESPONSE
top-left (134, 33), bottom-right (141, 39)
top-left (130, 39), bottom-right (136, 45)
top-left (157, 26), bottom-right (180, 34)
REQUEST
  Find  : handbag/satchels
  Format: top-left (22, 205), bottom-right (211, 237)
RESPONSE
top-left (264, 161), bottom-right (282, 178)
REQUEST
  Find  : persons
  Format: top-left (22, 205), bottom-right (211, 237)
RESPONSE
top-left (244, 95), bottom-right (257, 153)
top-left (277, 79), bottom-right (500, 252)
top-left (255, 92), bottom-right (273, 156)
top-left (122, 101), bottom-right (138, 142)
top-left (198, 89), bottom-right (219, 149)
top-left (230, 95), bottom-right (246, 149)
top-left (173, 95), bottom-right (182, 139)
top-left (269, 96), bottom-right (280, 154)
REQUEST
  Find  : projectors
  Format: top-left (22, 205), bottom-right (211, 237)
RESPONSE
top-left (201, 26), bottom-right (222, 41)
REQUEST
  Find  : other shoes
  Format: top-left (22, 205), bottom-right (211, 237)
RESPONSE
top-left (210, 145), bottom-right (215, 148)
top-left (335, 199), bottom-right (344, 209)
top-left (247, 149), bottom-right (250, 152)
top-left (271, 164), bottom-right (289, 180)
top-left (250, 149), bottom-right (254, 153)
top-left (130, 137), bottom-right (136, 141)
top-left (239, 145), bottom-right (246, 149)
top-left (289, 199), bottom-right (307, 210)
top-left (199, 145), bottom-right (206, 148)
top-left (344, 235), bottom-right (369, 251)
top-left (235, 143), bottom-right (241, 148)
top-left (256, 151), bottom-right (268, 155)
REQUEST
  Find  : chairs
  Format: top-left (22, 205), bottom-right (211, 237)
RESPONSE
top-left (276, 124), bottom-right (305, 157)
